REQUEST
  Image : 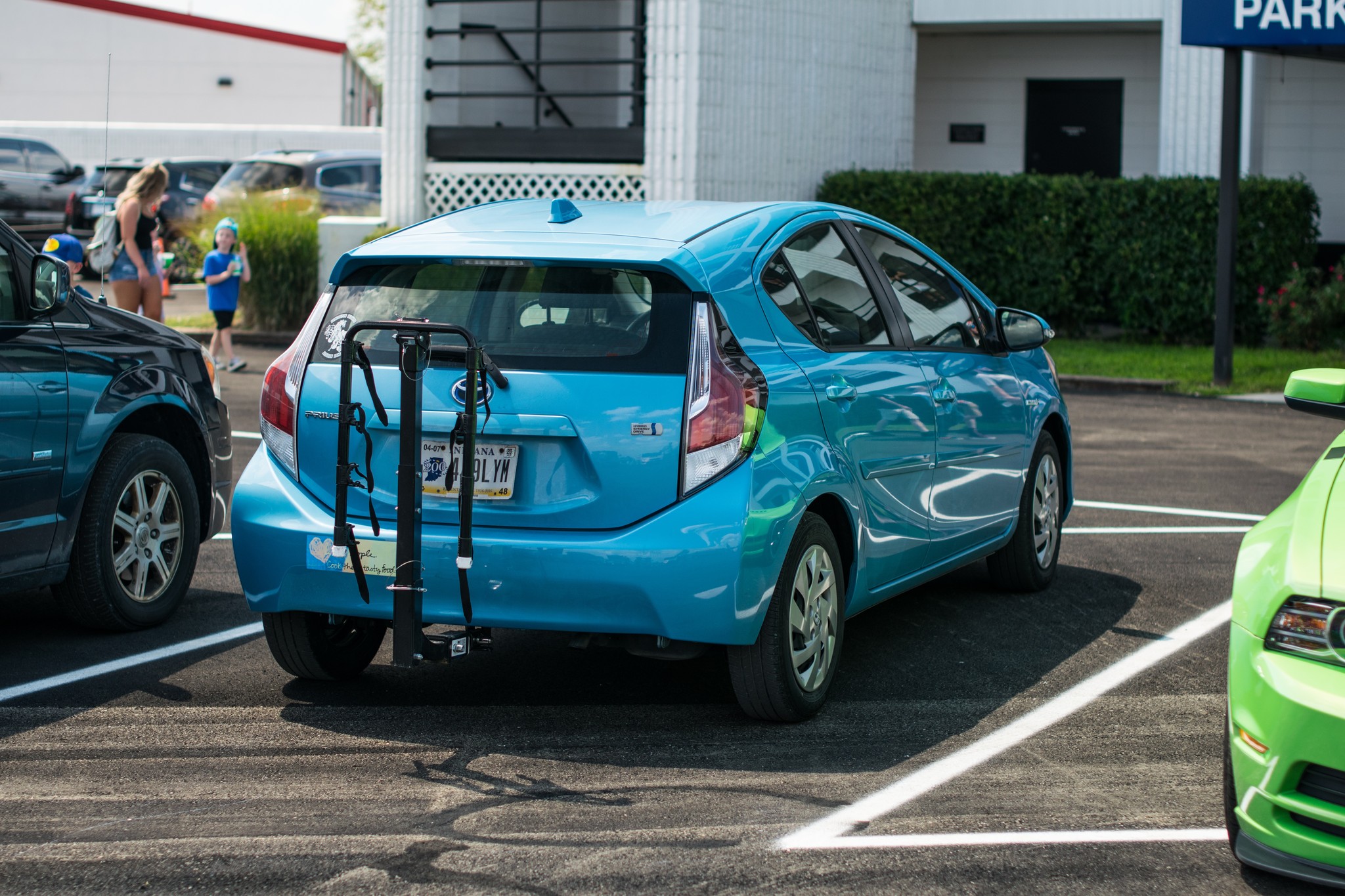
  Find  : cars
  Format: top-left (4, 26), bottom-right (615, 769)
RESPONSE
top-left (1223, 369), bottom-right (1345, 889)
top-left (0, 217), bottom-right (234, 635)
top-left (232, 198), bottom-right (1074, 727)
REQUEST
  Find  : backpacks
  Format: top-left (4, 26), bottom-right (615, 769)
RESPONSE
top-left (87, 195), bottom-right (143, 274)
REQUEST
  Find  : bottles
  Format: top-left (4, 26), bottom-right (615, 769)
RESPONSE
top-left (232, 260), bottom-right (242, 277)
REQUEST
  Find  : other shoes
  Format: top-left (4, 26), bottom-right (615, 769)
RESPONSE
top-left (211, 356), bottom-right (226, 370)
top-left (227, 356), bottom-right (246, 373)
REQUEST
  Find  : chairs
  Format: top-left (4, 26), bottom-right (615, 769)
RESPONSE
top-left (511, 265), bottom-right (640, 355)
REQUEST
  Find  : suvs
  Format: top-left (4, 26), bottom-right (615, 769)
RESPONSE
top-left (79, 154), bottom-right (234, 236)
top-left (201, 149), bottom-right (381, 220)
top-left (0, 134), bottom-right (89, 246)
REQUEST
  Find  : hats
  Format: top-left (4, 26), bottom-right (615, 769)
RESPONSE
top-left (213, 216), bottom-right (239, 252)
top-left (42, 233), bottom-right (83, 264)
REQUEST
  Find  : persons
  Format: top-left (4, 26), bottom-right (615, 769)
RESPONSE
top-left (39, 234), bottom-right (94, 300)
top-left (202, 217), bottom-right (248, 371)
top-left (105, 161), bottom-right (168, 325)
